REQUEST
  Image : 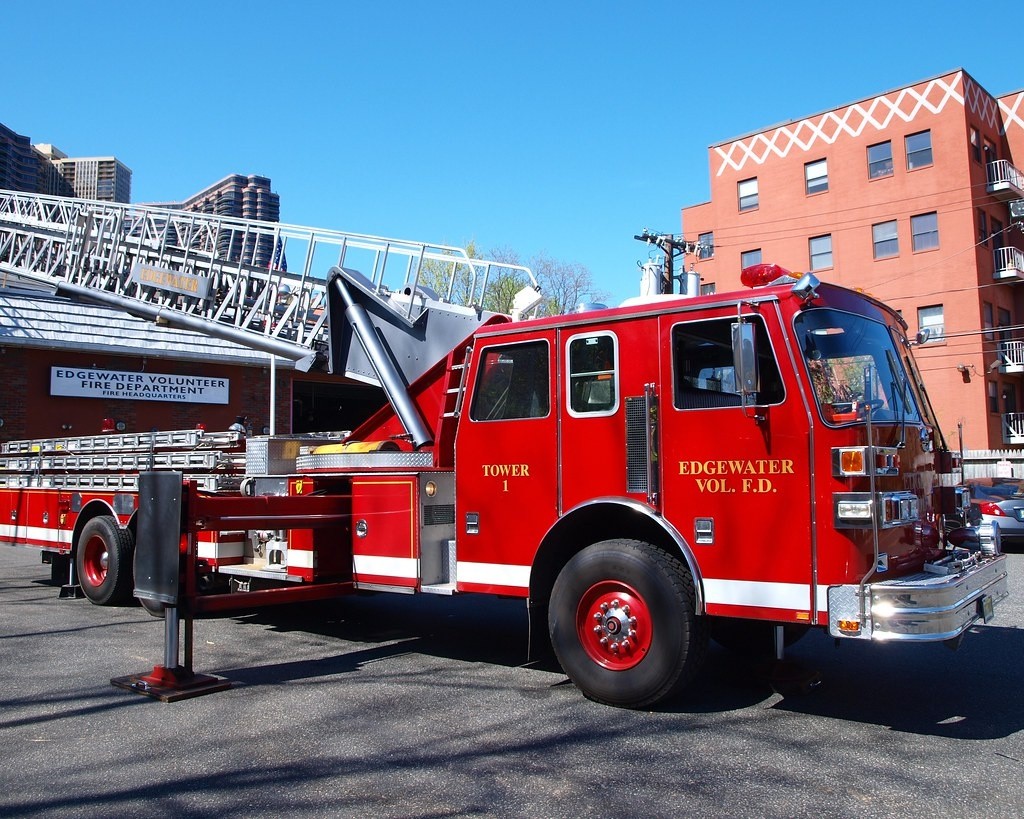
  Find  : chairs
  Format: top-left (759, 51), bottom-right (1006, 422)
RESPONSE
top-left (679, 387), bottom-right (742, 409)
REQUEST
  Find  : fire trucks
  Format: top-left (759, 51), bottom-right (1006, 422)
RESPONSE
top-left (0, 190), bottom-right (1011, 708)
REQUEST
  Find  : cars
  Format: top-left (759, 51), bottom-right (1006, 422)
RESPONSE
top-left (954, 477), bottom-right (1024, 546)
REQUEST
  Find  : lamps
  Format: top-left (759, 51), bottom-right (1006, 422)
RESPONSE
top-left (1002, 392), bottom-right (1006, 398)
top-left (990, 230), bottom-right (994, 236)
top-left (997, 322), bottom-right (1003, 328)
top-left (983, 144), bottom-right (988, 150)
top-left (957, 365), bottom-right (968, 372)
